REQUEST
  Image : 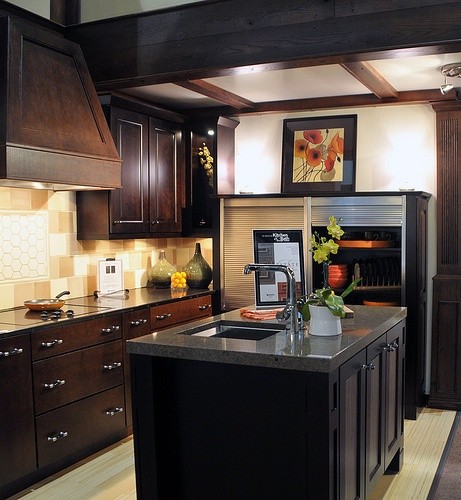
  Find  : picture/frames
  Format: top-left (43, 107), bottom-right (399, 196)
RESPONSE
top-left (280, 114), bottom-right (357, 192)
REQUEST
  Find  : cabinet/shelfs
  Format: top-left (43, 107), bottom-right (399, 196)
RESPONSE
top-left (30, 306), bottom-right (150, 484)
top-left (126, 320), bottom-right (407, 500)
top-left (110, 93), bottom-right (184, 239)
top-left (190, 123), bottom-right (217, 239)
top-left (0, 331), bottom-right (39, 500)
top-left (151, 292), bottom-right (213, 331)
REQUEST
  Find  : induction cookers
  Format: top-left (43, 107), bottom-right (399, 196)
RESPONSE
top-left (0, 300), bottom-right (112, 326)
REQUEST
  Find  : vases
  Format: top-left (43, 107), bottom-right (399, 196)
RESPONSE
top-left (184, 242), bottom-right (213, 292)
top-left (147, 250), bottom-right (176, 285)
top-left (307, 301), bottom-right (343, 336)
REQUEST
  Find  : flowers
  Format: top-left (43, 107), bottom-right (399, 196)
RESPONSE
top-left (199, 143), bottom-right (215, 190)
top-left (299, 214), bottom-right (363, 322)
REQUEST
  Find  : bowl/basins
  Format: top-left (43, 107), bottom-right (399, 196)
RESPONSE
top-left (363, 297), bottom-right (401, 306)
top-left (327, 264), bottom-right (348, 289)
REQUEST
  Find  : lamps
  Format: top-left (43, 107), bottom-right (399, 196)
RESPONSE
top-left (441, 64), bottom-right (461, 96)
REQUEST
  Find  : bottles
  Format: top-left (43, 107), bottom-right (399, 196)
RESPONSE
top-left (147, 250), bottom-right (177, 287)
top-left (183, 242), bottom-right (213, 288)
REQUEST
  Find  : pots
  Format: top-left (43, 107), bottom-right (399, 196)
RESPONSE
top-left (24, 290), bottom-right (70, 311)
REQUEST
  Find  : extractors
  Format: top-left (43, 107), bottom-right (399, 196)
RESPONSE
top-left (0, 14), bottom-right (123, 194)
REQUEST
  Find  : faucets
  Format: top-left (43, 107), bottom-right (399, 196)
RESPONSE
top-left (243, 262), bottom-right (298, 331)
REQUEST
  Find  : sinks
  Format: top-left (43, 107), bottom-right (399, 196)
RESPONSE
top-left (175, 319), bottom-right (286, 341)
